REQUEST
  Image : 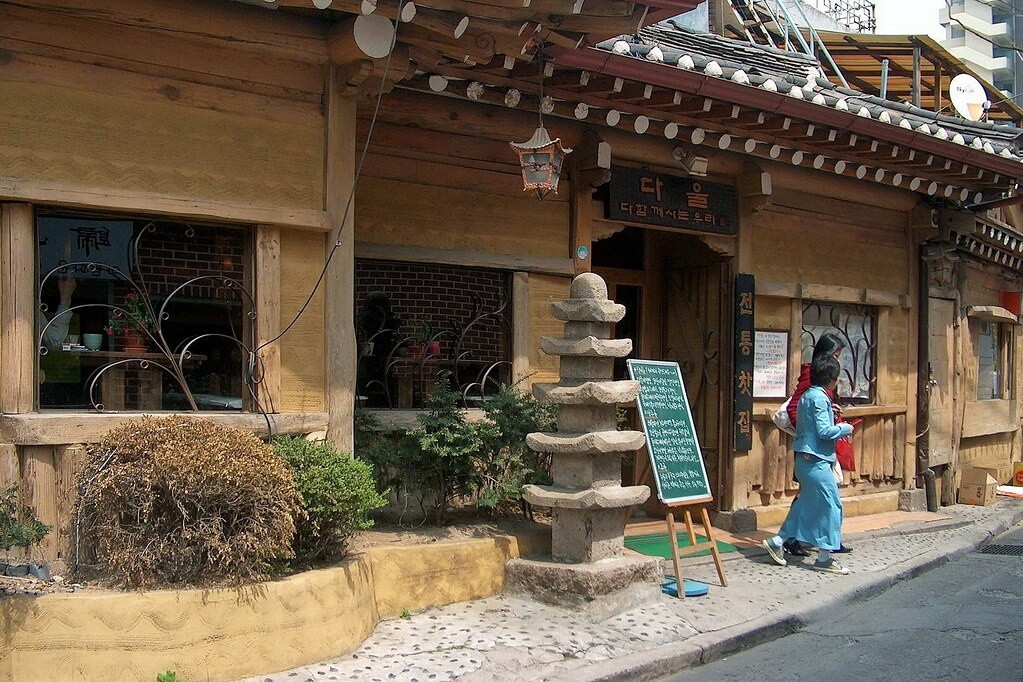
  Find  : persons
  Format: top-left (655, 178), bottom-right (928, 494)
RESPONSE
top-left (763, 333), bottom-right (854, 573)
top-left (37, 267), bottom-right (77, 354)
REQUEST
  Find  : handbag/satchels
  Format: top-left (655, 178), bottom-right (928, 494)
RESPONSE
top-left (835, 435), bottom-right (855, 471)
top-left (772, 395), bottom-right (797, 437)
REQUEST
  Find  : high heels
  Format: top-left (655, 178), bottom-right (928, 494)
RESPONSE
top-left (831, 543), bottom-right (853, 553)
top-left (783, 540), bottom-right (811, 556)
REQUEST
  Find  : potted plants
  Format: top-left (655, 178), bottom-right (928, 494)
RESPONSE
top-left (0, 499), bottom-right (50, 578)
top-left (422, 328), bottom-right (442, 355)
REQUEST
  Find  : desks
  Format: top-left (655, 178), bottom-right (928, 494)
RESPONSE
top-left (392, 357), bottom-right (497, 407)
top-left (70, 349), bottom-right (207, 410)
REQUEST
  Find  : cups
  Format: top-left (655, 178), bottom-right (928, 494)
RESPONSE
top-left (361, 342), bottom-right (374, 355)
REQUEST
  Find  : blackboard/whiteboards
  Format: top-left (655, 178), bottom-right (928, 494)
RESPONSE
top-left (627, 358), bottom-right (714, 507)
top-left (752, 328), bottom-right (792, 402)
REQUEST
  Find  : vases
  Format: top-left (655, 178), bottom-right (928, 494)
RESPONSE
top-left (123, 326), bottom-right (148, 355)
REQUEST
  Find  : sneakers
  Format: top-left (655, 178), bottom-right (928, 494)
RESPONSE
top-left (813, 558), bottom-right (849, 574)
top-left (763, 537), bottom-right (787, 566)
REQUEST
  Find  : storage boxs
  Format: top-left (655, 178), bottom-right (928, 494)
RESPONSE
top-left (957, 466), bottom-right (998, 505)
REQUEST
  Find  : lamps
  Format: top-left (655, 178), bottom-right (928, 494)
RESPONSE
top-left (509, 45), bottom-right (573, 201)
top-left (681, 150), bottom-right (707, 177)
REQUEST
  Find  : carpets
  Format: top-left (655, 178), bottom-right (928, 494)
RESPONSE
top-left (623, 529), bottom-right (744, 560)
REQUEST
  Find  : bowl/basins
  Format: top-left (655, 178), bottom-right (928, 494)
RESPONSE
top-left (65, 335), bottom-right (79, 344)
top-left (83, 334), bottom-right (102, 351)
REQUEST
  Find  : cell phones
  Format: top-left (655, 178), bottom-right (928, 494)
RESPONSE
top-left (849, 418), bottom-right (861, 426)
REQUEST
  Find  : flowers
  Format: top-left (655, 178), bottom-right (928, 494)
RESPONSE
top-left (105, 292), bottom-right (164, 337)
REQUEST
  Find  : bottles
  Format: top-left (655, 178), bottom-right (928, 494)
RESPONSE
top-left (202, 373), bottom-right (242, 397)
